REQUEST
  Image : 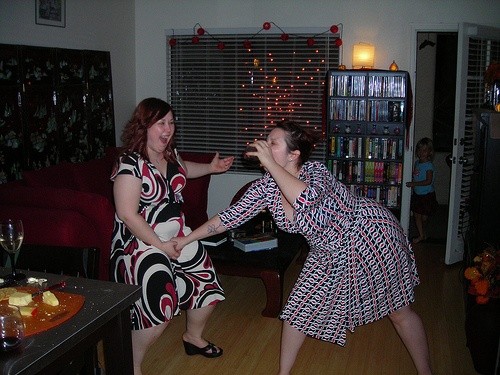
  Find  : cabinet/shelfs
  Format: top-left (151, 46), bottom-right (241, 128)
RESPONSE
top-left (325, 71), bottom-right (410, 223)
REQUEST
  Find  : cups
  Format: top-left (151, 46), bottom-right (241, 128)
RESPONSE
top-left (244, 142), bottom-right (266, 159)
top-left (0, 303), bottom-right (22, 353)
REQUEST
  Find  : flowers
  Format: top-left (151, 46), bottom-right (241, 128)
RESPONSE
top-left (463, 247), bottom-right (500, 305)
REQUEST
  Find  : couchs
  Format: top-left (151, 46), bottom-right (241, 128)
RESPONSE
top-left (0, 145), bottom-right (210, 281)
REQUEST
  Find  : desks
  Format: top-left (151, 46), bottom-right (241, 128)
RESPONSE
top-left (198, 226), bottom-right (308, 318)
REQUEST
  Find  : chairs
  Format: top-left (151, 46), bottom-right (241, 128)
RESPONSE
top-left (0, 244), bottom-right (100, 280)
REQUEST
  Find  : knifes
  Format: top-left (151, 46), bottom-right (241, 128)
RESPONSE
top-left (29, 280), bottom-right (69, 298)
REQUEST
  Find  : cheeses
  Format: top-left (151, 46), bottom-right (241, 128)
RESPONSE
top-left (9, 292), bottom-right (33, 306)
top-left (43, 291), bottom-right (59, 306)
top-left (19, 307), bottom-right (37, 317)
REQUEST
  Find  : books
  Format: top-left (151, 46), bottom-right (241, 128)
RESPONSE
top-left (329, 75), bottom-right (405, 207)
top-left (201, 231), bottom-right (277, 252)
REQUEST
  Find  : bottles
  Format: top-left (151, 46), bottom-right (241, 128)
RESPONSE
top-left (394, 127), bottom-right (401, 135)
top-left (356, 126), bottom-right (362, 134)
top-left (334, 124), bottom-right (341, 134)
top-left (383, 126), bottom-right (390, 135)
top-left (344, 125), bottom-right (352, 134)
top-left (371, 124), bottom-right (377, 134)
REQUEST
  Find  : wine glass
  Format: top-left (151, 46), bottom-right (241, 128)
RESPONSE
top-left (0, 216), bottom-right (28, 281)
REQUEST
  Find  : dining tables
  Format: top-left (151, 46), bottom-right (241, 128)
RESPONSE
top-left (0, 267), bottom-right (143, 375)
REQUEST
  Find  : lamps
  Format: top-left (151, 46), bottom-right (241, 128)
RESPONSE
top-left (352, 43), bottom-right (375, 69)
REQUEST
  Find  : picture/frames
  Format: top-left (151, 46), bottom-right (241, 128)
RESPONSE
top-left (35, 0), bottom-right (66, 28)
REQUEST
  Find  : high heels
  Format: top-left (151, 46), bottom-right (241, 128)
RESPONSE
top-left (185, 340), bottom-right (223, 357)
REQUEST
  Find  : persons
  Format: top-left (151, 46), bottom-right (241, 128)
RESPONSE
top-left (168, 121), bottom-right (434, 375)
top-left (110, 98), bottom-right (235, 375)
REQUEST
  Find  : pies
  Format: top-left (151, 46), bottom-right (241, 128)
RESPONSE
top-left (0, 288), bottom-right (17, 302)
